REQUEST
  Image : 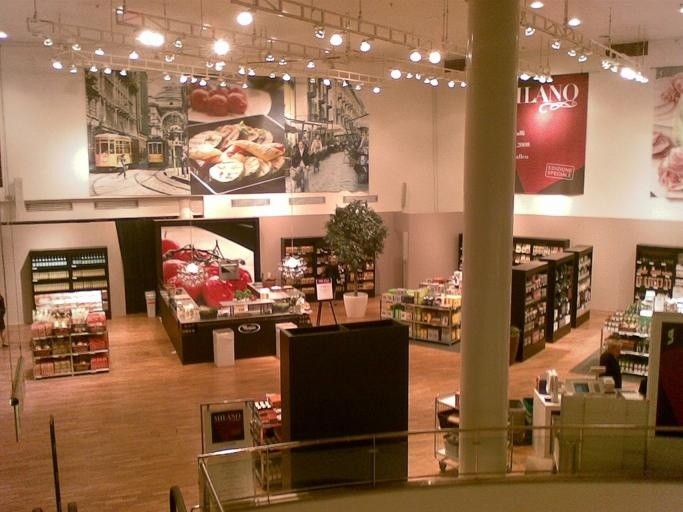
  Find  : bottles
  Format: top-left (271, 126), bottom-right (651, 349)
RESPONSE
top-left (31, 252), bottom-right (105, 268)
top-left (422, 296), bottom-right (433, 306)
top-left (623, 302), bottom-right (640, 325)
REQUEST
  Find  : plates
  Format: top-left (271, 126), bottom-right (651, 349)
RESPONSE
top-left (650, 157), bottom-right (683, 200)
top-left (186, 113), bottom-right (284, 194)
top-left (653, 76), bottom-right (678, 127)
top-left (162, 226), bottom-right (253, 282)
top-left (187, 80), bottom-right (272, 123)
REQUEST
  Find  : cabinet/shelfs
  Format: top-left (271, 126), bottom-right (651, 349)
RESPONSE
top-left (68, 301), bottom-right (111, 377)
top-left (615, 327), bottom-right (650, 380)
top-left (277, 317), bottom-right (409, 492)
top-left (631, 242), bottom-right (682, 305)
top-left (29, 316), bottom-right (72, 381)
top-left (457, 233), bottom-right (463, 273)
top-left (377, 288), bottom-right (416, 342)
top-left (529, 387), bottom-right (643, 461)
top-left (415, 286), bottom-right (461, 347)
top-left (27, 247), bottom-right (72, 315)
top-left (539, 250), bottom-right (574, 343)
top-left (511, 236), bottom-right (531, 266)
top-left (280, 235), bottom-right (317, 304)
top-left (563, 244), bottom-right (593, 329)
top-left (344, 255), bottom-right (375, 300)
top-left (248, 397), bottom-right (283, 493)
top-left (598, 318), bottom-right (615, 357)
top-left (531, 236), bottom-right (570, 259)
top-left (512, 258), bottom-right (547, 362)
top-left (68, 246), bottom-right (112, 322)
top-left (312, 236), bottom-right (346, 300)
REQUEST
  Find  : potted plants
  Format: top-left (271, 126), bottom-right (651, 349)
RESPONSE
top-left (509, 324), bottom-right (519, 366)
top-left (322, 197), bottom-right (391, 318)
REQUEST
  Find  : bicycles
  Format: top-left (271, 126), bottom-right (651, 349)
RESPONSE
top-left (289, 167), bottom-right (310, 192)
top-left (311, 153), bottom-right (320, 172)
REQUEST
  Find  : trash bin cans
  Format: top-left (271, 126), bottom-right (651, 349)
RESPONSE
top-left (212, 327), bottom-right (237, 368)
top-left (508, 399), bottom-right (534, 447)
top-left (275, 322), bottom-right (299, 358)
top-left (143, 289), bottom-right (156, 318)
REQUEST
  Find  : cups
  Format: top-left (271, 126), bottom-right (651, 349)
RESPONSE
top-left (635, 338), bottom-right (649, 353)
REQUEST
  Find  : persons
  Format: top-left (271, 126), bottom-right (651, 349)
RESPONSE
top-left (0, 294), bottom-right (9, 347)
top-left (324, 255), bottom-right (343, 297)
top-left (288, 132), bottom-right (361, 193)
top-left (600, 339), bottom-right (623, 389)
top-left (118, 154), bottom-right (128, 180)
top-left (179, 151), bottom-right (189, 178)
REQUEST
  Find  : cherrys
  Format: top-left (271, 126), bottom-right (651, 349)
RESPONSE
top-left (163, 239), bottom-right (252, 310)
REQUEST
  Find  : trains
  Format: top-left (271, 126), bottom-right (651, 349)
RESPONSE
top-left (93, 134), bottom-right (141, 173)
top-left (145, 138), bottom-right (167, 167)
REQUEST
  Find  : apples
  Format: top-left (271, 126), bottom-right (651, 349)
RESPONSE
top-left (191, 86), bottom-right (248, 116)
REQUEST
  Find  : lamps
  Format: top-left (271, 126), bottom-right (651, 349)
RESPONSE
top-left (0, 0), bottom-right (655, 93)
top-left (174, 210), bottom-right (210, 289)
